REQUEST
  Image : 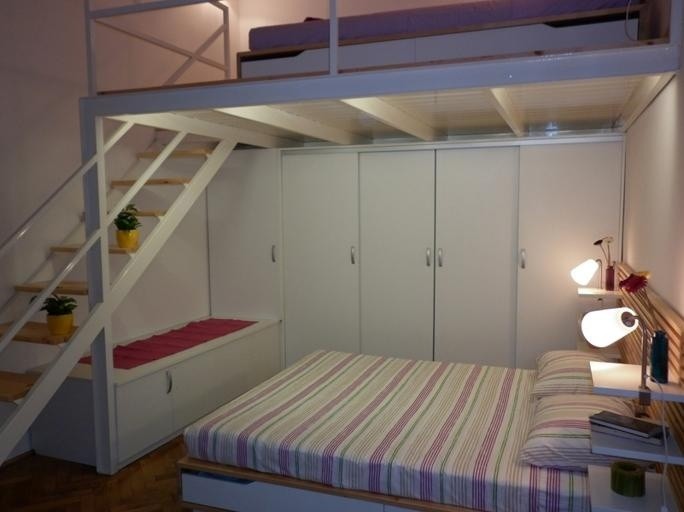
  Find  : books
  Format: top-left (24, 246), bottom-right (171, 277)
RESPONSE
top-left (590, 422), bottom-right (669, 446)
top-left (588, 409), bottom-right (670, 438)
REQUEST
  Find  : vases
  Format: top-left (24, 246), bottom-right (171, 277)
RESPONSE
top-left (606, 263), bottom-right (614, 292)
top-left (651, 330), bottom-right (669, 382)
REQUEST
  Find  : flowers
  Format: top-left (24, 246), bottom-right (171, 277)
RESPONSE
top-left (593, 235), bottom-right (614, 265)
top-left (619, 272), bottom-right (676, 337)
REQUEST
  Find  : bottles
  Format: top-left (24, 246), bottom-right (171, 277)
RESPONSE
top-left (604, 266), bottom-right (614, 291)
top-left (649, 330), bottom-right (669, 385)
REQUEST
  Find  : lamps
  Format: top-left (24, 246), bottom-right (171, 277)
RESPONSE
top-left (580, 307), bottom-right (648, 391)
top-left (569, 259), bottom-right (603, 290)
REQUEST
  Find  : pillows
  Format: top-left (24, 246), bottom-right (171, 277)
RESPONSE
top-left (516, 394), bottom-right (652, 472)
top-left (533, 349), bottom-right (622, 396)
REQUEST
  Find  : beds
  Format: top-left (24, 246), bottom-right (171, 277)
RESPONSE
top-left (233, 0), bottom-right (671, 80)
top-left (176, 257), bottom-right (684, 512)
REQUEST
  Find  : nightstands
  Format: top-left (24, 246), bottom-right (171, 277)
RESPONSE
top-left (578, 288), bottom-right (620, 359)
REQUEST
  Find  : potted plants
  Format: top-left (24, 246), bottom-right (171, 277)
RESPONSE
top-left (27, 290), bottom-right (77, 337)
top-left (113, 204), bottom-right (143, 248)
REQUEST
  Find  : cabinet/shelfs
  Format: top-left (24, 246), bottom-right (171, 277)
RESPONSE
top-left (204, 147), bottom-right (282, 385)
top-left (589, 360), bottom-right (684, 512)
top-left (518, 135), bottom-right (625, 367)
top-left (283, 145), bottom-right (359, 375)
top-left (358, 141), bottom-right (519, 375)
top-left (23, 316), bottom-right (282, 473)
